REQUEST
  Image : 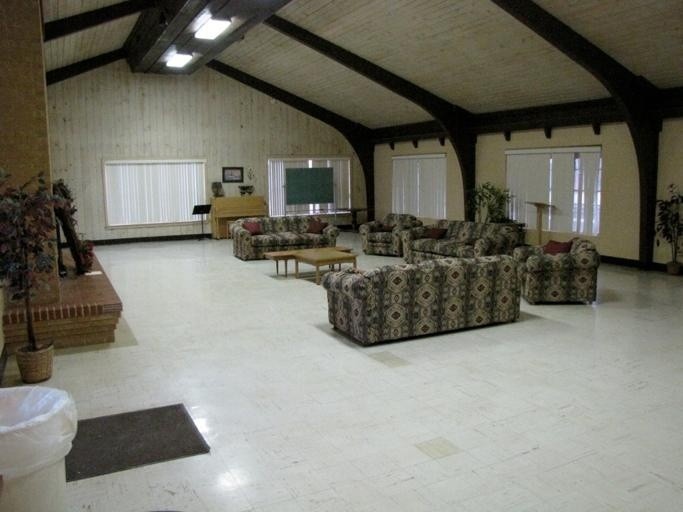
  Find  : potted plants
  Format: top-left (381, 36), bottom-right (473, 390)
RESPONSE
top-left (655, 183), bottom-right (683, 275)
top-left (0, 169), bottom-right (68, 384)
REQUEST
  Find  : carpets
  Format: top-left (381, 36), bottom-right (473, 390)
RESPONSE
top-left (65, 403), bottom-right (210, 485)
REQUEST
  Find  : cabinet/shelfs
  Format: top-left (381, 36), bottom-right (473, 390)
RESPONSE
top-left (210, 195), bottom-right (268, 240)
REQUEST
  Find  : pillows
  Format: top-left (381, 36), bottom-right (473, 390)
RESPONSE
top-left (543, 239), bottom-right (572, 256)
top-left (242, 222), bottom-right (264, 235)
top-left (422, 228), bottom-right (448, 239)
top-left (307, 220), bottom-right (328, 234)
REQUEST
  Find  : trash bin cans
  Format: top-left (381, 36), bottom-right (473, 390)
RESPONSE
top-left (0, 385), bottom-right (77, 512)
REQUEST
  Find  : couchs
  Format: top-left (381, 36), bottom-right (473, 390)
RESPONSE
top-left (229, 215), bottom-right (340, 261)
top-left (400, 219), bottom-right (519, 265)
top-left (358, 213), bottom-right (423, 258)
top-left (512, 237), bottom-right (601, 305)
top-left (321, 254), bottom-right (523, 346)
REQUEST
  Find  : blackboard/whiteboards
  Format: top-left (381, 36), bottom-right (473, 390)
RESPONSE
top-left (283, 166), bottom-right (335, 206)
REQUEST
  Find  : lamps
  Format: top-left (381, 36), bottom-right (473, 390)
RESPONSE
top-left (193, 16), bottom-right (232, 40)
top-left (166, 52), bottom-right (193, 68)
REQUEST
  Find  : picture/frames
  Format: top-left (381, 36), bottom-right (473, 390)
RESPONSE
top-left (222, 167), bottom-right (244, 183)
